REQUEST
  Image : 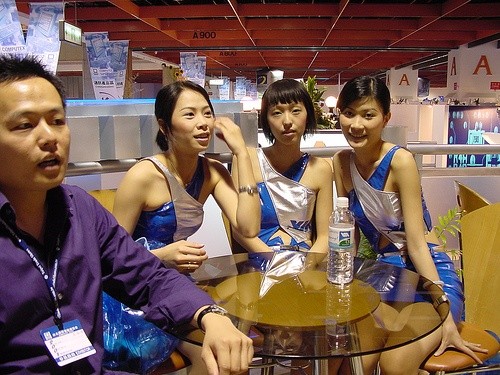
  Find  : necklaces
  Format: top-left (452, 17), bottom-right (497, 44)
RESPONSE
top-left (168, 154), bottom-right (196, 187)
top-left (362, 159), bottom-right (372, 178)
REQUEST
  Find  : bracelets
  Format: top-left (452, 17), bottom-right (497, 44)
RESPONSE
top-left (238, 186), bottom-right (259, 195)
top-left (423, 280), bottom-right (445, 289)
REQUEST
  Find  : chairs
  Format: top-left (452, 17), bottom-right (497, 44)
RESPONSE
top-left (420, 178), bottom-right (500, 375)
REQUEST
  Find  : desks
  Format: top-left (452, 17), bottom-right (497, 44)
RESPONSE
top-left (153, 255), bottom-right (450, 375)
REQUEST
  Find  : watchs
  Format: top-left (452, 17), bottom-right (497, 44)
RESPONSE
top-left (197, 304), bottom-right (228, 335)
top-left (433, 295), bottom-right (450, 309)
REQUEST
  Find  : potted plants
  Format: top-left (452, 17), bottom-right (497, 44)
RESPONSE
top-left (300, 75), bottom-right (327, 124)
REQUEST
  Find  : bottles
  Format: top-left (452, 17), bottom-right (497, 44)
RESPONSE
top-left (327, 197), bottom-right (355, 285)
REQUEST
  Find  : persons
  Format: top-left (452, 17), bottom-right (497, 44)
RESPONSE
top-left (103, 80), bottom-right (262, 375)
top-left (332, 75), bottom-right (488, 375)
top-left (0, 52), bottom-right (254, 375)
top-left (230, 78), bottom-right (345, 375)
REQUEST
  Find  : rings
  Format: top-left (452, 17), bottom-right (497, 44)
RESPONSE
top-left (188, 264), bottom-right (190, 269)
